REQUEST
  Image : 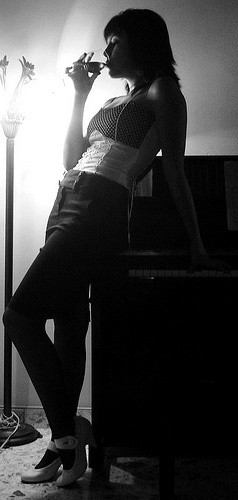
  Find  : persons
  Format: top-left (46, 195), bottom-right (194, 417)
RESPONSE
top-left (2, 8), bottom-right (212, 487)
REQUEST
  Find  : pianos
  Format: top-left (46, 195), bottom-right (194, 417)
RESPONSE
top-left (91, 155), bottom-right (238, 500)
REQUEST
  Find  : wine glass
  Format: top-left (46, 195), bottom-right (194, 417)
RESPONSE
top-left (73, 47), bottom-right (110, 80)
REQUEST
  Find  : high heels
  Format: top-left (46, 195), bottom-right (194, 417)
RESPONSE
top-left (21, 438), bottom-right (63, 482)
top-left (55, 416), bottom-right (97, 487)
